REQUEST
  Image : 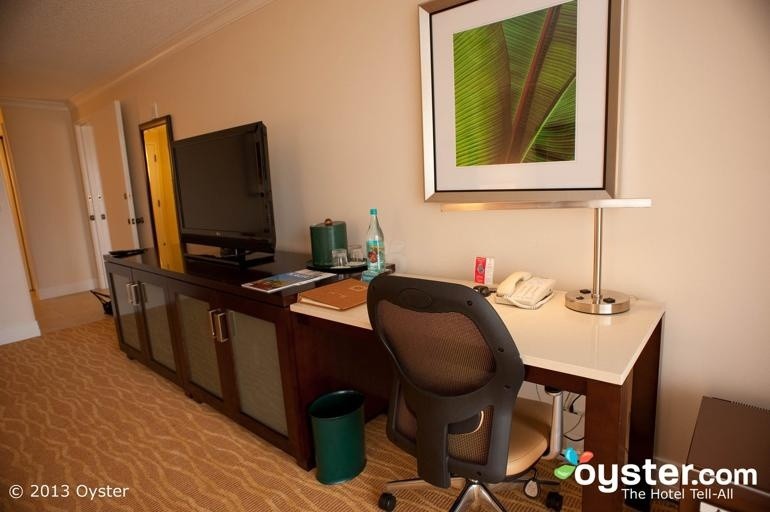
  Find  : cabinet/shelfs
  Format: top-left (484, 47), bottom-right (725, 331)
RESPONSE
top-left (102, 255), bottom-right (391, 470)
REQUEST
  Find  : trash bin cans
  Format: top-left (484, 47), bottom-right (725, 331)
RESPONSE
top-left (307, 391), bottom-right (366, 485)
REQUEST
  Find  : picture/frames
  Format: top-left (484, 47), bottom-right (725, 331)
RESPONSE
top-left (417, 0), bottom-right (624, 203)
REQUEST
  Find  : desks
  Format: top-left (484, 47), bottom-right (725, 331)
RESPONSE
top-left (289, 271), bottom-right (665, 512)
top-left (679, 396), bottom-right (770, 512)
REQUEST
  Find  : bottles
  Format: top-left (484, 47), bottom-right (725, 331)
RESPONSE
top-left (366, 208), bottom-right (386, 271)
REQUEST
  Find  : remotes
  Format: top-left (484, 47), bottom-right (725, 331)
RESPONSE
top-left (109, 248), bottom-right (146, 256)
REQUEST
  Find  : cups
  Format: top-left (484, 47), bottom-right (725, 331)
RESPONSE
top-left (331, 244), bottom-right (364, 267)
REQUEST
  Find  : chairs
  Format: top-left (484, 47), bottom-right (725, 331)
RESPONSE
top-left (366, 273), bottom-right (564, 512)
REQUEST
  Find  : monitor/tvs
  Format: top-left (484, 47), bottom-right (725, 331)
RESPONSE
top-left (168, 120), bottom-right (277, 265)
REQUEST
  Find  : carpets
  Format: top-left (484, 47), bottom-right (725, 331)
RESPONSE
top-left (0, 317), bottom-right (680, 512)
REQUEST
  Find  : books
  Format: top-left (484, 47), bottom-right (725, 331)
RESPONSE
top-left (295, 277), bottom-right (371, 312)
top-left (294, 267), bottom-right (339, 286)
top-left (242, 271), bottom-right (319, 293)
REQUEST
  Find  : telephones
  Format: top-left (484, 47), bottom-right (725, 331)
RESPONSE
top-left (494, 271), bottom-right (555, 306)
